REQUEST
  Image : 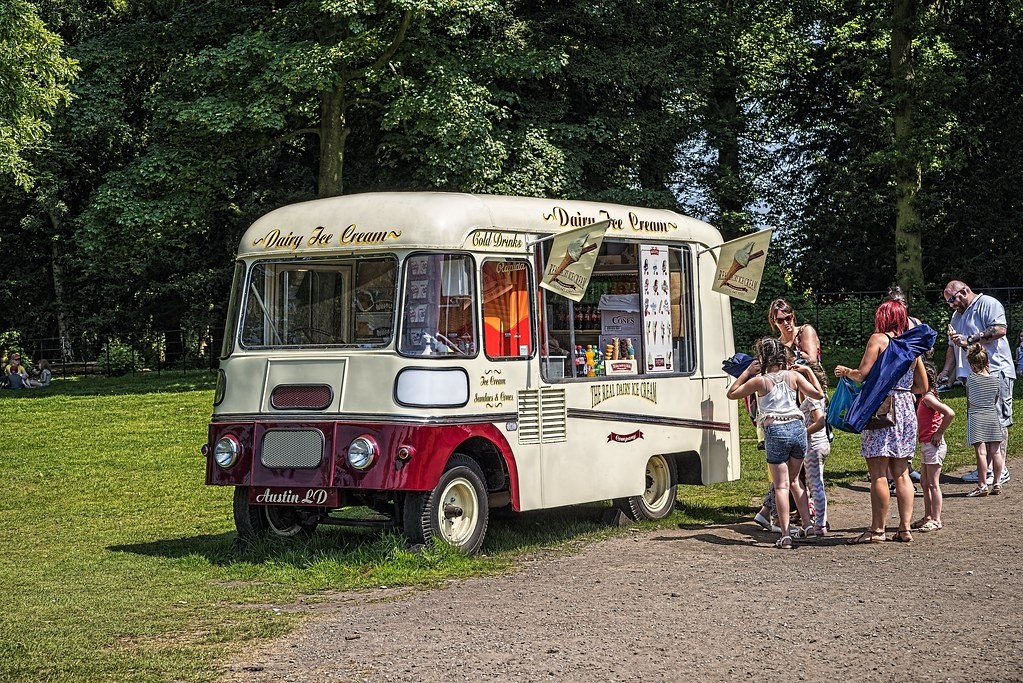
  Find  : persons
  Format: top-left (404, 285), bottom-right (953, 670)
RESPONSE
top-left (798, 361), bottom-right (831, 536)
top-left (936, 280), bottom-right (1016, 487)
top-left (1016, 331), bottom-right (1023, 376)
top-left (767, 298), bottom-right (820, 525)
top-left (834, 301), bottom-right (929, 544)
top-left (754, 480), bottom-right (800, 534)
top-left (966, 343), bottom-right (1001, 498)
top-left (911, 360), bottom-right (955, 532)
top-left (888, 288), bottom-right (921, 494)
top-left (727, 337), bottom-right (824, 549)
top-left (0, 353), bottom-right (51, 389)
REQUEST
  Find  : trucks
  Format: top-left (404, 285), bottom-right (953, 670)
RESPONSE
top-left (203, 191), bottom-right (741, 556)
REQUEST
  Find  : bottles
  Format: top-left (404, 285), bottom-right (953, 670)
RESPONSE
top-left (570, 344), bottom-right (602, 377)
top-left (551, 275), bottom-right (638, 304)
top-left (552, 304), bottom-right (601, 330)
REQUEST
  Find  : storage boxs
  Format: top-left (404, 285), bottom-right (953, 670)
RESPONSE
top-left (607, 254), bottom-right (627, 264)
top-left (670, 272), bottom-right (682, 304)
top-left (541, 355), bottom-right (568, 379)
top-left (597, 335), bottom-right (642, 373)
top-left (597, 255), bottom-right (607, 263)
top-left (672, 304), bottom-right (685, 338)
top-left (599, 292), bottom-right (640, 335)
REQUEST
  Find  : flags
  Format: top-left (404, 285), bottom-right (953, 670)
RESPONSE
top-left (538, 218), bottom-right (610, 302)
top-left (712, 227), bottom-right (773, 303)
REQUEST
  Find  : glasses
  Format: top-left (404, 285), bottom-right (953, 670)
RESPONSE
top-left (775, 315), bottom-right (793, 324)
top-left (947, 287), bottom-right (965, 304)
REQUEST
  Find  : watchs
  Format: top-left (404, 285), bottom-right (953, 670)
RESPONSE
top-left (967, 335), bottom-right (973, 345)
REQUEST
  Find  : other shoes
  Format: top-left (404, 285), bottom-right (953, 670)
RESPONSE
top-left (754, 513), bottom-right (772, 531)
top-left (910, 518), bottom-right (942, 532)
top-left (814, 527), bottom-right (827, 535)
top-left (887, 479), bottom-right (917, 496)
top-left (909, 470), bottom-right (921, 479)
top-left (771, 524), bottom-right (799, 534)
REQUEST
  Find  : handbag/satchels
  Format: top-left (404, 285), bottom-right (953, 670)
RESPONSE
top-left (864, 394), bottom-right (896, 430)
top-left (825, 375), bottom-right (865, 434)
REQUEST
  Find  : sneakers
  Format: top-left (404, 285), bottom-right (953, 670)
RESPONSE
top-left (986, 466), bottom-right (1011, 486)
top-left (961, 468), bottom-right (992, 482)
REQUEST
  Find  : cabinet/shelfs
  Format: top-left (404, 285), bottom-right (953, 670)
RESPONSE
top-left (546, 264), bottom-right (685, 341)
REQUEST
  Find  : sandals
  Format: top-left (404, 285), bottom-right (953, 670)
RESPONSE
top-left (966, 483), bottom-right (1002, 497)
top-left (793, 526), bottom-right (817, 543)
top-left (892, 530), bottom-right (913, 542)
top-left (776, 536), bottom-right (792, 549)
top-left (847, 529), bottom-right (886, 544)
